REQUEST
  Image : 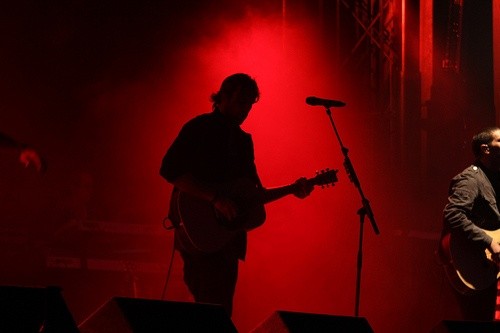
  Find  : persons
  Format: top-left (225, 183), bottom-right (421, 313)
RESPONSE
top-left (159, 74), bottom-right (314, 313)
top-left (443, 127), bottom-right (500, 323)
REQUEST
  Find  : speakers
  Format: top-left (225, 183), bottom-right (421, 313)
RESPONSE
top-left (77, 297), bottom-right (238, 333)
top-left (431, 320), bottom-right (500, 333)
top-left (0, 286), bottom-right (78, 333)
top-left (251, 310), bottom-right (376, 333)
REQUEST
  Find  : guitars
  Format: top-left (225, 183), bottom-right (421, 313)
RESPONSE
top-left (439, 227), bottom-right (500, 297)
top-left (168, 165), bottom-right (339, 257)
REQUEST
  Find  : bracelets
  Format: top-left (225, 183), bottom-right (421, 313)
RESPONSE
top-left (207, 191), bottom-right (223, 207)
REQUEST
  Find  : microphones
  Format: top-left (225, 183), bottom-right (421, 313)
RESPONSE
top-left (306, 96), bottom-right (347, 107)
